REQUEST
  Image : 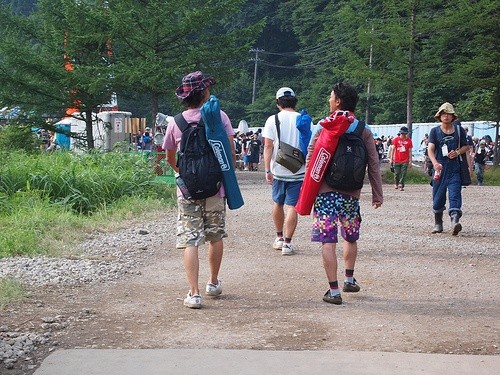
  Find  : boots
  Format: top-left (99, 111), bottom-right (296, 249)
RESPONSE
top-left (477, 179), bottom-right (482, 186)
top-left (431, 213), bottom-right (443, 233)
top-left (449, 213), bottom-right (462, 235)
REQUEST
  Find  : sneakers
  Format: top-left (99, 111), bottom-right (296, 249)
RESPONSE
top-left (184, 289), bottom-right (202, 308)
top-left (274, 237), bottom-right (284, 249)
top-left (342, 277), bottom-right (360, 292)
top-left (323, 289), bottom-right (341, 304)
top-left (282, 243), bottom-right (294, 255)
top-left (206, 280), bottom-right (222, 295)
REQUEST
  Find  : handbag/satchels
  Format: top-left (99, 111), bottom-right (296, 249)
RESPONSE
top-left (276, 143), bottom-right (305, 173)
top-left (460, 159), bottom-right (471, 186)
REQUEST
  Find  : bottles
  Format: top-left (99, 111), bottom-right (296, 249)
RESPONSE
top-left (391, 164), bottom-right (395, 173)
top-left (432, 163), bottom-right (442, 183)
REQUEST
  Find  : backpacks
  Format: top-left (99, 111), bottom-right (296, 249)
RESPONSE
top-left (172, 114), bottom-right (224, 199)
top-left (326, 120), bottom-right (368, 192)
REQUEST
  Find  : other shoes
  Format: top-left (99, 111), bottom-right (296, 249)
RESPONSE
top-left (400, 183), bottom-right (405, 191)
top-left (244, 168), bottom-right (249, 171)
top-left (395, 181), bottom-right (398, 188)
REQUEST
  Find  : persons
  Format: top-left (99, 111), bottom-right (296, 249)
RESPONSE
top-left (233, 129), bottom-right (265, 171)
top-left (162, 71), bottom-right (236, 308)
top-left (306, 83), bottom-right (383, 304)
top-left (130, 125), bottom-right (166, 152)
top-left (392, 127), bottom-right (412, 191)
top-left (374, 135), bottom-right (395, 173)
top-left (464, 127), bottom-right (500, 186)
top-left (36, 127), bottom-right (56, 151)
top-left (262, 86), bottom-right (313, 256)
top-left (420, 133), bottom-right (429, 174)
top-left (427, 102), bottom-right (468, 235)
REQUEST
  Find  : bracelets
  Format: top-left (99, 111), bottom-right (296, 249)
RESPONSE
top-left (265, 170), bottom-right (271, 174)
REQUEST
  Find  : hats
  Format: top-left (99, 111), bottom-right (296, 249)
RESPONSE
top-left (275, 87), bottom-right (295, 99)
top-left (480, 140), bottom-right (485, 145)
top-left (434, 102), bottom-right (457, 122)
top-left (399, 127), bottom-right (408, 134)
top-left (175, 70), bottom-right (216, 100)
